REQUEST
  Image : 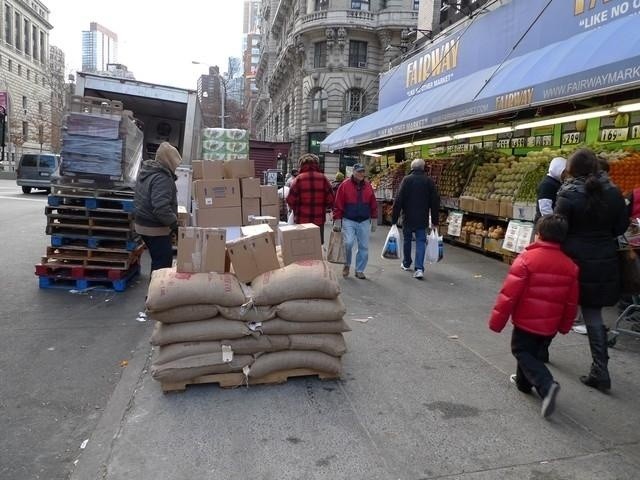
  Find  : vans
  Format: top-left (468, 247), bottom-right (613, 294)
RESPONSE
top-left (16, 152), bottom-right (61, 193)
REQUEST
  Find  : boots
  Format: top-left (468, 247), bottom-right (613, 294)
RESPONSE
top-left (578, 324), bottom-right (612, 391)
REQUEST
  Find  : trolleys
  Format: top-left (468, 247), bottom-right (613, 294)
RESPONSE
top-left (604, 216), bottom-right (639, 349)
top-left (263, 167), bottom-right (288, 224)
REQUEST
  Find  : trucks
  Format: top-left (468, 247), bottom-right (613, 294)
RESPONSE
top-left (71, 70), bottom-right (206, 247)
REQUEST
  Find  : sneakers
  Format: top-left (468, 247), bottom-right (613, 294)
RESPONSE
top-left (400, 262), bottom-right (411, 271)
top-left (540, 381), bottom-right (560, 418)
top-left (412, 268), bottom-right (424, 278)
top-left (342, 266), bottom-right (366, 279)
top-left (509, 374), bottom-right (517, 385)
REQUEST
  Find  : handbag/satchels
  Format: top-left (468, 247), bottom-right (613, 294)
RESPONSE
top-left (327, 228), bottom-right (348, 264)
top-left (424, 225), bottom-right (444, 265)
top-left (381, 223), bottom-right (401, 260)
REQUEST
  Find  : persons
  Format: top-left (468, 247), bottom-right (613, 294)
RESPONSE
top-left (488, 212), bottom-right (582, 418)
top-left (392, 158), bottom-right (440, 279)
top-left (334, 163), bottom-right (378, 279)
top-left (133, 141), bottom-right (181, 276)
top-left (284, 151), bottom-right (334, 247)
top-left (535, 155), bottom-right (590, 334)
top-left (286, 168), bottom-right (299, 186)
top-left (556, 148), bottom-right (630, 393)
top-left (332, 172), bottom-right (345, 191)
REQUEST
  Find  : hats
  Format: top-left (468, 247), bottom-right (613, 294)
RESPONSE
top-left (352, 163), bottom-right (366, 172)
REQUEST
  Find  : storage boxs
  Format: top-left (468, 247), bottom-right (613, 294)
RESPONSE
top-left (176, 154), bottom-right (323, 282)
top-left (439, 194), bottom-right (534, 258)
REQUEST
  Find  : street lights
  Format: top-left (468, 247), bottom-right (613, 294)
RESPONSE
top-left (190, 60), bottom-right (228, 130)
